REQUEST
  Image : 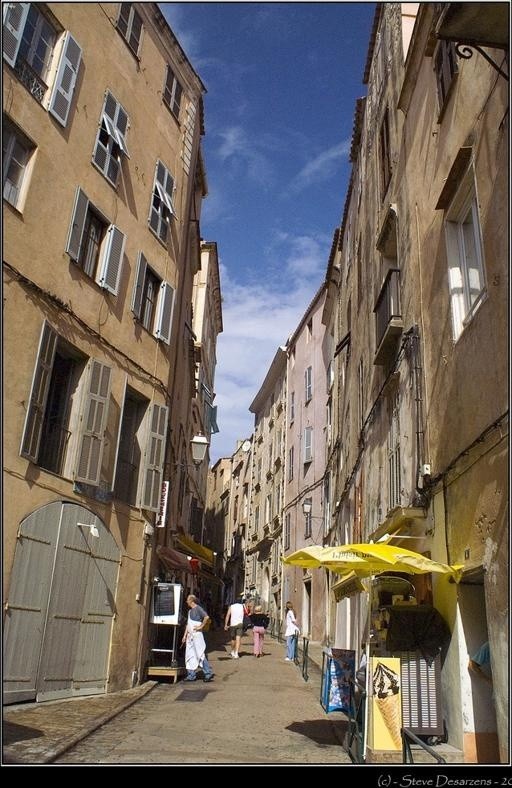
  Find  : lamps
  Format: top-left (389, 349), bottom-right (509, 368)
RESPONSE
top-left (166, 427), bottom-right (209, 478)
top-left (75, 521), bottom-right (99, 538)
top-left (301, 497), bottom-right (323, 524)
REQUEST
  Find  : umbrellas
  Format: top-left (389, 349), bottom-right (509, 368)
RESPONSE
top-left (277, 540), bottom-right (468, 761)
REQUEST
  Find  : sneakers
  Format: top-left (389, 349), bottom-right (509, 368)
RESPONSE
top-left (255, 652), bottom-right (265, 658)
top-left (284, 656), bottom-right (294, 661)
top-left (231, 650), bottom-right (240, 659)
top-left (183, 674), bottom-right (215, 682)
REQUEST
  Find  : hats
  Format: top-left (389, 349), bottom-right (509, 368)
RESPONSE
top-left (253, 605), bottom-right (264, 615)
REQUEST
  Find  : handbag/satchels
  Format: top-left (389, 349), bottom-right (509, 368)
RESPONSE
top-left (243, 604), bottom-right (251, 632)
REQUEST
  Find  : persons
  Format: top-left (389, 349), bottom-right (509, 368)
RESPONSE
top-left (181, 594), bottom-right (216, 683)
top-left (283, 600), bottom-right (301, 661)
top-left (470, 641), bottom-right (496, 703)
top-left (224, 596), bottom-right (249, 659)
top-left (242, 598), bottom-right (252, 631)
top-left (248, 603), bottom-right (270, 657)
